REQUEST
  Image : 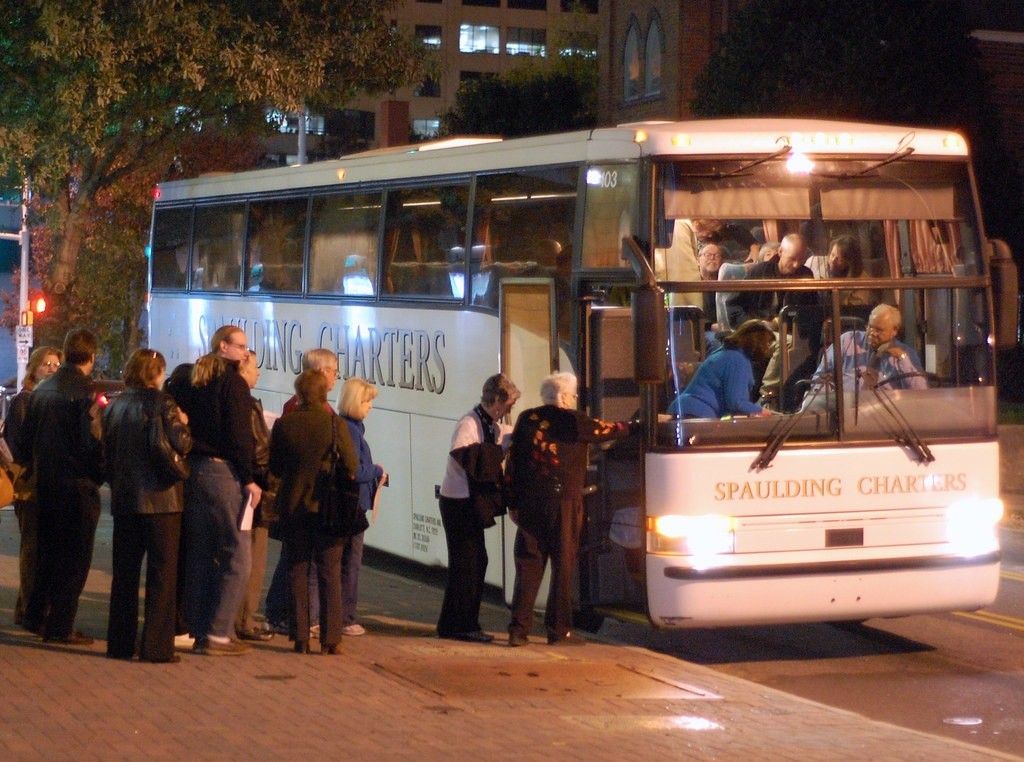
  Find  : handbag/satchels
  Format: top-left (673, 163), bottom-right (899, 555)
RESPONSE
top-left (0, 464), bottom-right (30, 509)
top-left (149, 393), bottom-right (191, 484)
top-left (318, 414), bottom-right (368, 537)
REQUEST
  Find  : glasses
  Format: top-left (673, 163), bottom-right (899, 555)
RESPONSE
top-left (237, 344), bottom-right (249, 351)
top-left (574, 395), bottom-right (578, 399)
top-left (42, 362), bottom-right (60, 368)
top-left (704, 253), bottom-right (722, 259)
top-left (326, 369), bottom-right (340, 377)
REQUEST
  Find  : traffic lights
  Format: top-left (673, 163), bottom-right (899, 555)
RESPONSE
top-left (33, 299), bottom-right (47, 317)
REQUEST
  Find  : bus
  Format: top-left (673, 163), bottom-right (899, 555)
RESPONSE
top-left (145, 117), bottom-right (1018, 631)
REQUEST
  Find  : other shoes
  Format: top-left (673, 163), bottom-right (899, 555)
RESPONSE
top-left (452, 631), bottom-right (493, 642)
top-left (42, 627), bottom-right (94, 644)
top-left (192, 635), bottom-right (248, 655)
top-left (547, 630), bottom-right (585, 646)
top-left (509, 634), bottom-right (528, 645)
top-left (310, 625), bottom-right (320, 638)
top-left (238, 627), bottom-right (274, 640)
top-left (342, 624), bottom-right (365, 635)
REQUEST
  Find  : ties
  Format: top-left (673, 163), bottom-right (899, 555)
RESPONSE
top-left (861, 351), bottom-right (880, 391)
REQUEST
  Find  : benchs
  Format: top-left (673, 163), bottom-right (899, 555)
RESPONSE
top-left (715, 263), bottom-right (756, 332)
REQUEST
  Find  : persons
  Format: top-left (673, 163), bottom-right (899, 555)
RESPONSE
top-left (505, 372), bottom-right (641, 646)
top-left (163, 324), bottom-right (389, 656)
top-left (696, 219), bottom-right (878, 361)
top-left (810, 303), bottom-right (928, 394)
top-left (102, 347), bottom-right (192, 663)
top-left (667, 319), bottom-right (773, 420)
top-left (437, 374), bottom-right (521, 642)
top-left (3, 329), bottom-right (108, 647)
